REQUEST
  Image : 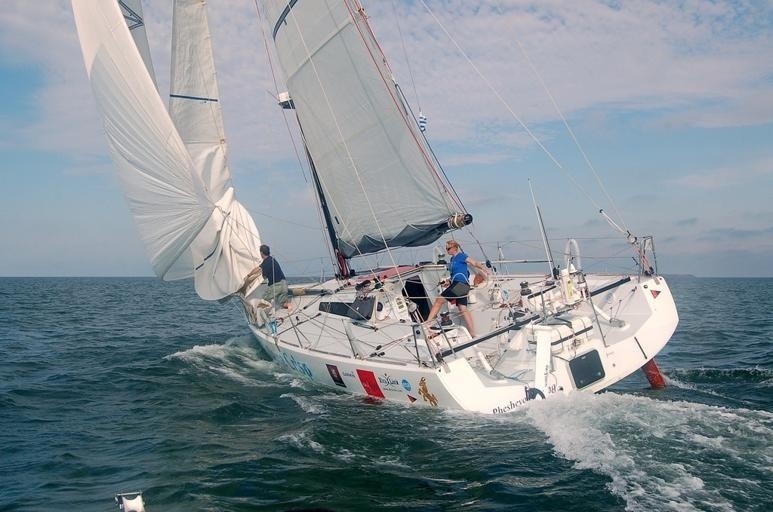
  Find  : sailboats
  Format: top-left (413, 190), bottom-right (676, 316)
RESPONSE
top-left (71, 0), bottom-right (680, 415)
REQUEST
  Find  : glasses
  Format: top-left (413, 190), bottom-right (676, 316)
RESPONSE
top-left (446, 246), bottom-right (454, 251)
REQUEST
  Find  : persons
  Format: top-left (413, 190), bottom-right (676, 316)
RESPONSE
top-left (418, 238), bottom-right (493, 340)
top-left (345, 280), bottom-right (375, 326)
top-left (241, 244), bottom-right (296, 321)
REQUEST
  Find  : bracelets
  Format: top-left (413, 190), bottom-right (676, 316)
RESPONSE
top-left (247, 274), bottom-right (250, 277)
